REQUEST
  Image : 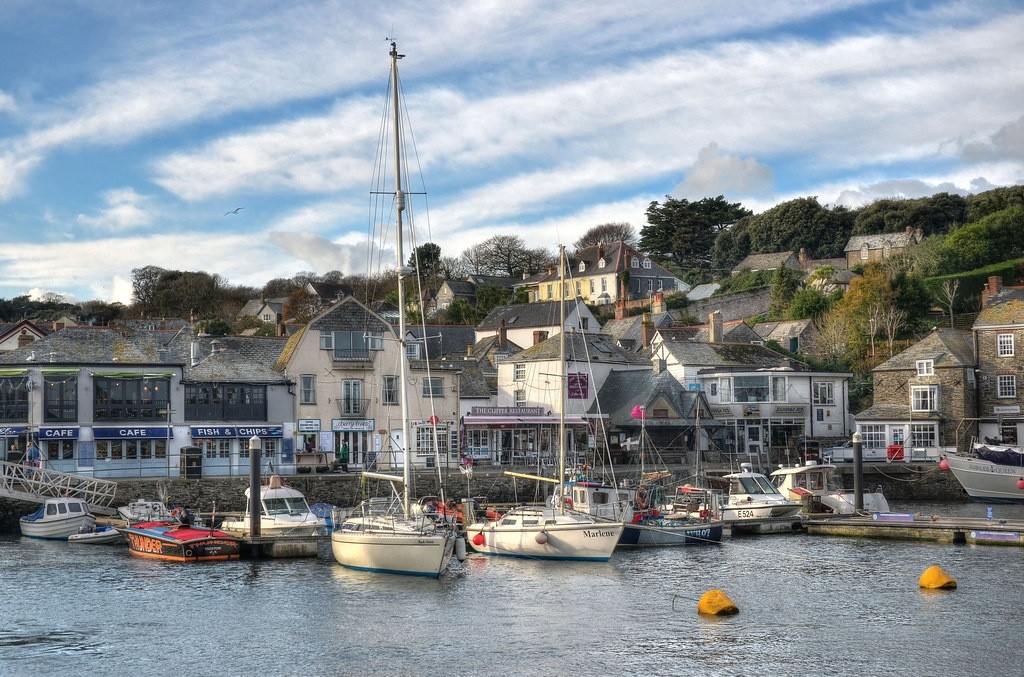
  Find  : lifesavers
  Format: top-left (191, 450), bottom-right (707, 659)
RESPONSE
top-left (170, 507), bottom-right (183, 516)
top-left (636, 487), bottom-right (650, 508)
top-left (557, 499), bottom-right (574, 508)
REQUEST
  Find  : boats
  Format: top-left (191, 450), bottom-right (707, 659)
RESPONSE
top-left (18, 498), bottom-right (96, 541)
top-left (115, 518), bottom-right (241, 562)
top-left (64, 525), bottom-right (121, 546)
top-left (768, 459), bottom-right (890, 513)
top-left (117, 499), bottom-right (172, 523)
top-left (932, 444), bottom-right (1024, 505)
top-left (220, 477), bottom-right (319, 534)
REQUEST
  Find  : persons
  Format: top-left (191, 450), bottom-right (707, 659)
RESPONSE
top-left (27, 442), bottom-right (39, 466)
top-left (304, 437), bottom-right (314, 453)
top-left (984, 436), bottom-right (1004, 445)
top-left (339, 440), bottom-right (349, 473)
top-left (10, 445), bottom-right (17, 451)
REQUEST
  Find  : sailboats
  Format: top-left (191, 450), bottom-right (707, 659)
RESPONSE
top-left (596, 404), bottom-right (723, 546)
top-left (550, 404), bottom-right (633, 516)
top-left (684, 396), bottom-right (804, 518)
top-left (331, 35), bottom-right (463, 577)
top-left (465, 244), bottom-right (626, 562)
